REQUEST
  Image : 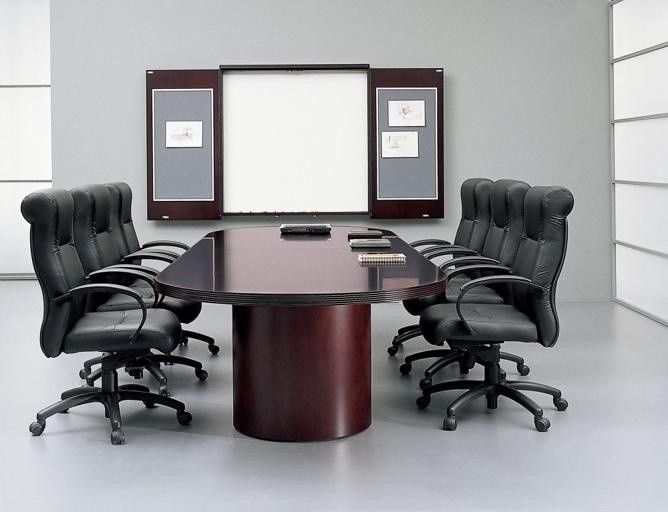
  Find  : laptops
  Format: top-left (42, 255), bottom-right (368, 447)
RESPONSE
top-left (279, 223), bottom-right (332, 235)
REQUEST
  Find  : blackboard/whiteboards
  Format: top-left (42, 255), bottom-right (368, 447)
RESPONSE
top-left (218, 64), bottom-right (372, 218)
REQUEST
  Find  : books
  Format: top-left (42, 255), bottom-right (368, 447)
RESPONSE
top-left (348, 231), bottom-right (383, 239)
top-left (358, 253), bottom-right (407, 263)
top-left (350, 239), bottom-right (392, 247)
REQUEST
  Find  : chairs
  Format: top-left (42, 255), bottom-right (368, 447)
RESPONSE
top-left (21, 188), bottom-right (192, 446)
top-left (398, 181), bottom-right (530, 389)
top-left (385, 178), bottom-right (503, 357)
top-left (104, 180), bottom-right (220, 364)
top-left (72, 186), bottom-right (208, 397)
top-left (416, 186), bottom-right (574, 435)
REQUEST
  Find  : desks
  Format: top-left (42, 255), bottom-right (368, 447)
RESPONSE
top-left (156, 223), bottom-right (446, 442)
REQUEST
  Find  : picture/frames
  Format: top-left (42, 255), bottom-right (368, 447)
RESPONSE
top-left (165, 121), bottom-right (204, 149)
top-left (386, 98), bottom-right (428, 127)
top-left (381, 130), bottom-right (420, 160)
